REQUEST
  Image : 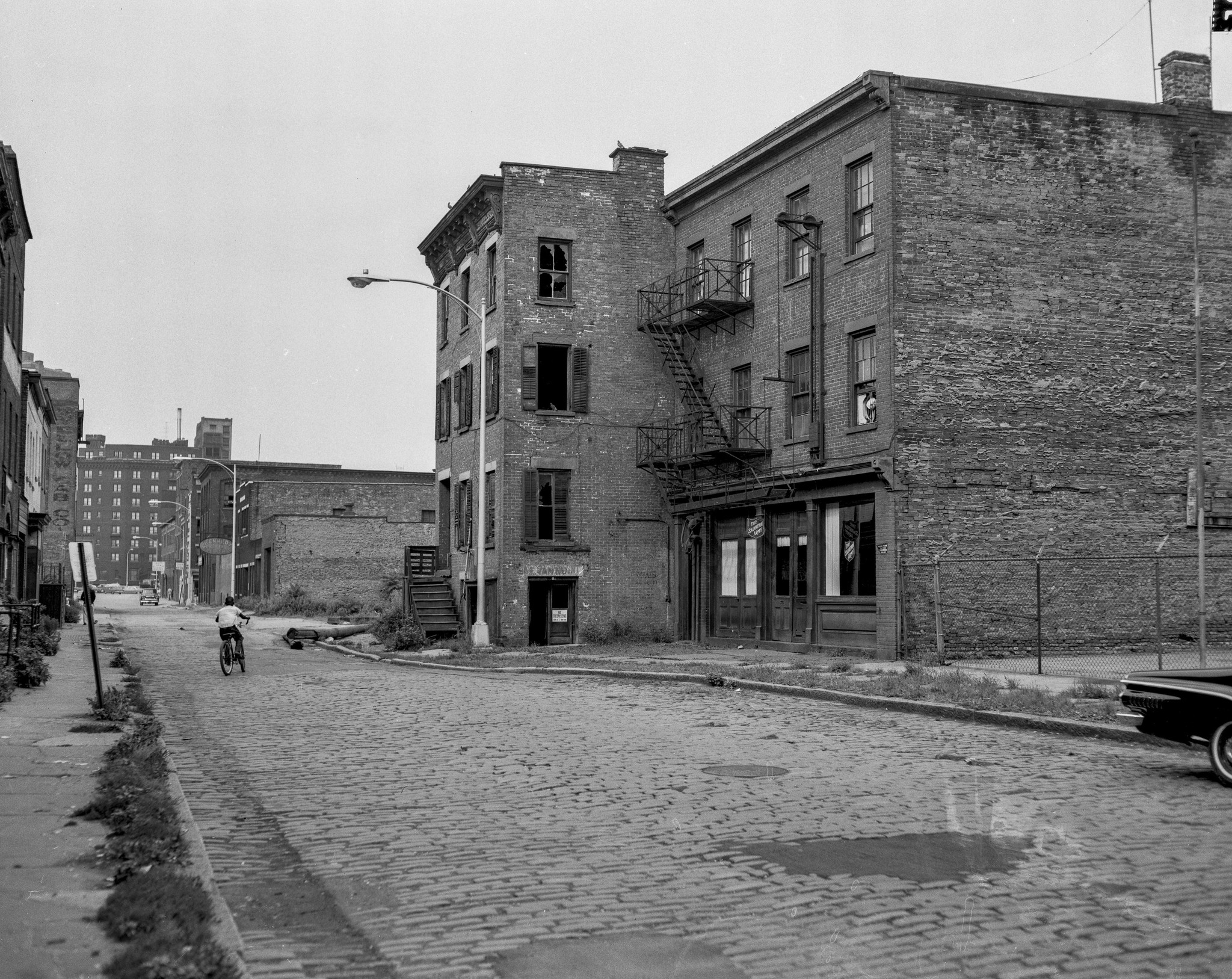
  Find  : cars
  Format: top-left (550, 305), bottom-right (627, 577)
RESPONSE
top-left (1114, 667), bottom-right (1232, 787)
top-left (76, 590), bottom-right (84, 600)
top-left (138, 588), bottom-right (160, 606)
top-left (74, 582), bottom-right (161, 595)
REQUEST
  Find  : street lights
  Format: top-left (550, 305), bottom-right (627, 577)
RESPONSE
top-left (172, 457), bottom-right (237, 605)
top-left (148, 500), bottom-right (192, 606)
top-left (152, 522), bottom-right (184, 605)
top-left (126, 548), bottom-right (134, 587)
top-left (132, 536), bottom-right (158, 589)
top-left (347, 269), bottom-right (490, 648)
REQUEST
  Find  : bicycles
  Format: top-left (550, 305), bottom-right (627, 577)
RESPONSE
top-left (219, 618), bottom-right (250, 676)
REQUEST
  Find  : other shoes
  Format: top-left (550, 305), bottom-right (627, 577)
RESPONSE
top-left (223, 657), bottom-right (229, 664)
top-left (235, 652), bottom-right (245, 659)
top-left (94, 620), bottom-right (98, 624)
top-left (83, 623), bottom-right (87, 625)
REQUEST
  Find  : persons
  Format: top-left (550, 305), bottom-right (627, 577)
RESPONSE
top-left (81, 584), bottom-right (98, 625)
top-left (215, 596), bottom-right (250, 664)
top-left (167, 587), bottom-right (172, 601)
